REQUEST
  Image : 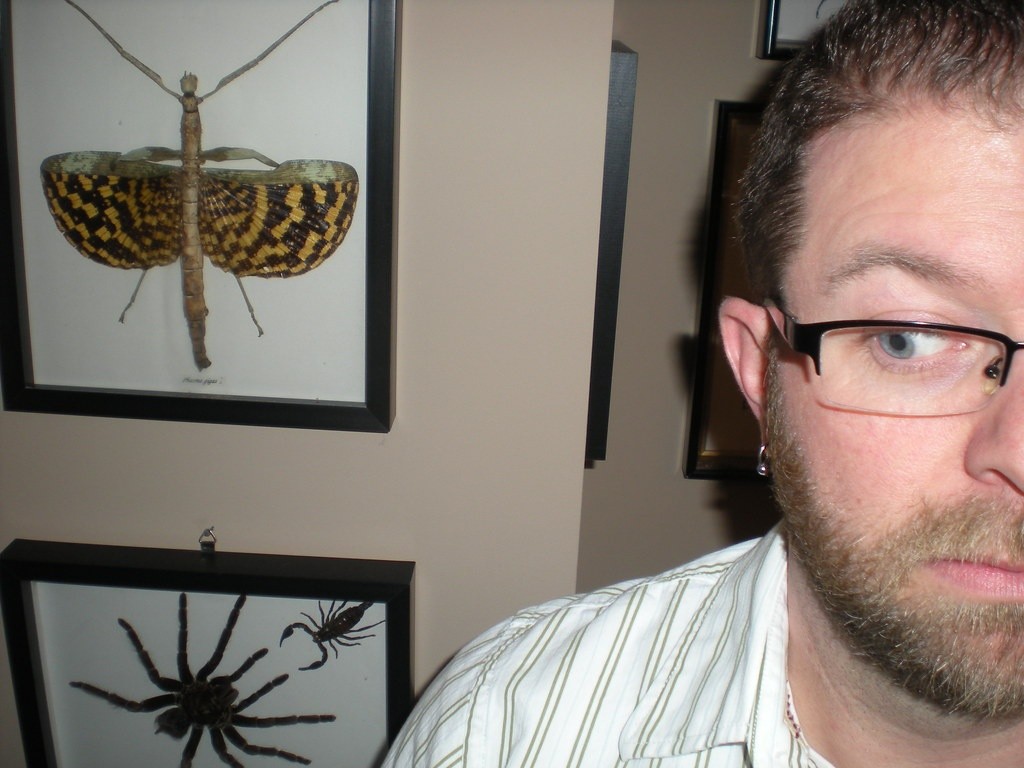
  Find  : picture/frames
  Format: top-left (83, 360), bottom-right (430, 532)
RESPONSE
top-left (0, 0), bottom-right (402, 432)
top-left (0, 538), bottom-right (415, 768)
top-left (755, 0), bottom-right (852, 60)
top-left (683, 97), bottom-right (772, 480)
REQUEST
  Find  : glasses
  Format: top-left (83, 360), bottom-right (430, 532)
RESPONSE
top-left (764, 297), bottom-right (1024, 416)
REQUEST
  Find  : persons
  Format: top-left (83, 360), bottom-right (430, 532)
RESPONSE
top-left (380, 0), bottom-right (1024, 768)
